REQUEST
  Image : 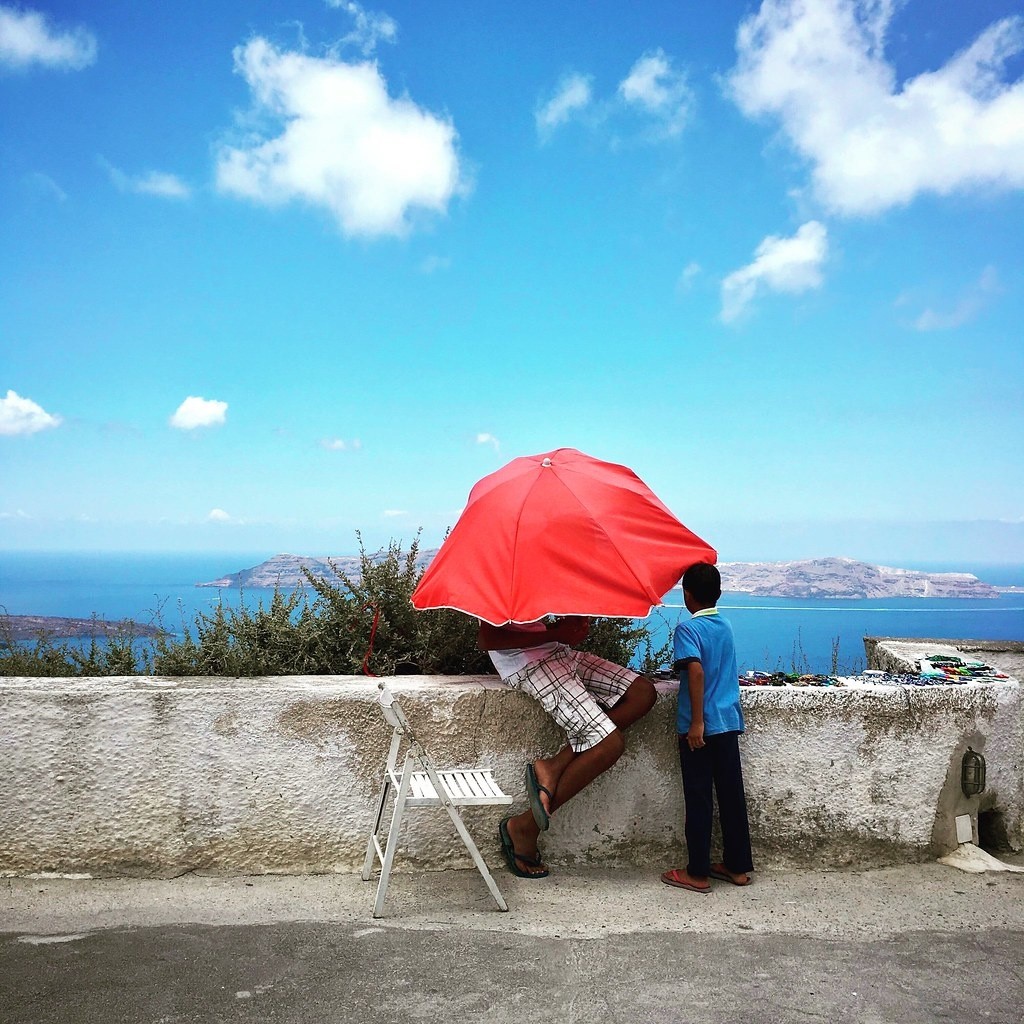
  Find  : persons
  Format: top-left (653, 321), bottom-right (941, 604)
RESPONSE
top-left (476, 617), bottom-right (656, 879)
top-left (661, 564), bottom-right (754, 893)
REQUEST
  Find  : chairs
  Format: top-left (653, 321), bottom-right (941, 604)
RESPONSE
top-left (362, 682), bottom-right (514, 919)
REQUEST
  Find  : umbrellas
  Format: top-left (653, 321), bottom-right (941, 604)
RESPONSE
top-left (410, 448), bottom-right (719, 627)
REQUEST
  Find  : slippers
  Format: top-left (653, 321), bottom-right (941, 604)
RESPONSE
top-left (661, 870), bottom-right (712, 893)
top-left (526, 762), bottom-right (554, 832)
top-left (709, 863), bottom-right (751, 885)
top-left (499, 816), bottom-right (549, 879)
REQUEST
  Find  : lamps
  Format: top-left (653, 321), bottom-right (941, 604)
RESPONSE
top-left (961, 746), bottom-right (986, 799)
